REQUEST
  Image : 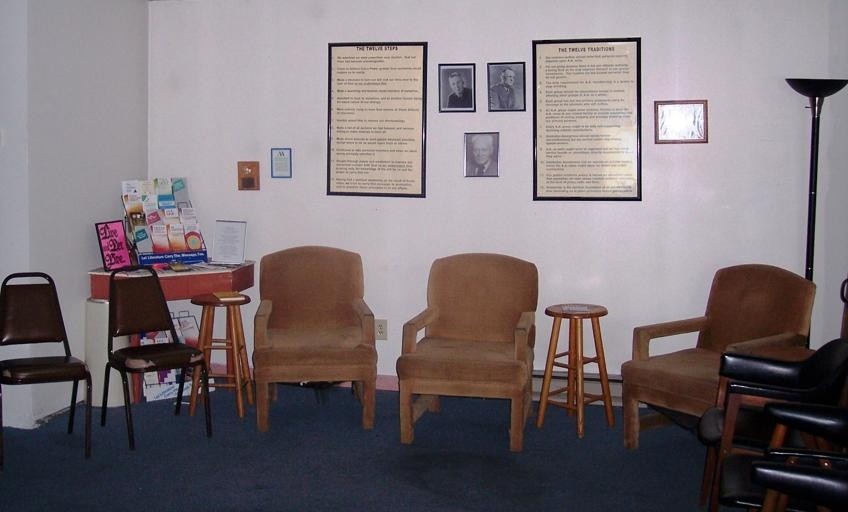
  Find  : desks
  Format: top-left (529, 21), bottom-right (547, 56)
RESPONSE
top-left (90, 262), bottom-right (254, 405)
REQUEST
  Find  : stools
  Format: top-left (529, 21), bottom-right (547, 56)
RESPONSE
top-left (535, 304), bottom-right (619, 440)
top-left (187, 290), bottom-right (255, 420)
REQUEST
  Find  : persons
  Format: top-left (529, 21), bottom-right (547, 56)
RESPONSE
top-left (446, 72), bottom-right (473, 108)
top-left (490, 68), bottom-right (516, 110)
top-left (469, 134), bottom-right (497, 176)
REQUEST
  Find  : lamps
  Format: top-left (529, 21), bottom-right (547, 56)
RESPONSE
top-left (784, 71), bottom-right (845, 290)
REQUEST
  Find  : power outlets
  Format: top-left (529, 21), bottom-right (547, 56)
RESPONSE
top-left (374, 317), bottom-right (387, 341)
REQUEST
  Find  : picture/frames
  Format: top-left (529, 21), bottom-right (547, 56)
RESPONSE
top-left (327, 39), bottom-right (428, 198)
top-left (653, 100), bottom-right (708, 144)
top-left (463, 131), bottom-right (498, 178)
top-left (437, 62), bottom-right (474, 113)
top-left (486, 60), bottom-right (525, 112)
top-left (532, 34), bottom-right (647, 203)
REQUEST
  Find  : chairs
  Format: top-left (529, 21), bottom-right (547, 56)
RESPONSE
top-left (621, 260), bottom-right (817, 450)
top-left (100, 265), bottom-right (214, 449)
top-left (398, 252), bottom-right (542, 455)
top-left (719, 401), bottom-right (848, 512)
top-left (253, 246), bottom-right (378, 433)
top-left (698, 280), bottom-right (847, 512)
top-left (0, 270), bottom-right (94, 458)
